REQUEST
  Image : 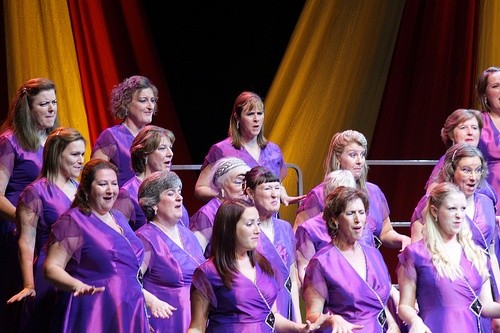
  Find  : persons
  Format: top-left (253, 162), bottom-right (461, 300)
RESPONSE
top-left (42, 159), bottom-right (151, 333)
top-left (476, 67), bottom-right (500, 235)
top-left (186, 198), bottom-right (333, 333)
top-left (90, 76), bottom-right (158, 190)
top-left (396, 182), bottom-right (500, 333)
top-left (410, 144), bottom-right (500, 333)
top-left (195, 91), bottom-right (307, 219)
top-left (302, 186), bottom-right (400, 333)
top-left (296, 170), bottom-right (407, 326)
top-left (0, 78), bottom-right (59, 333)
top-left (242, 166), bottom-right (302, 333)
top-left (293, 130), bottom-right (411, 251)
top-left (424, 109), bottom-right (500, 270)
top-left (189, 156), bottom-right (252, 254)
top-left (112, 125), bottom-right (189, 232)
top-left (7, 127), bottom-right (86, 333)
top-left (134, 170), bottom-right (206, 333)
